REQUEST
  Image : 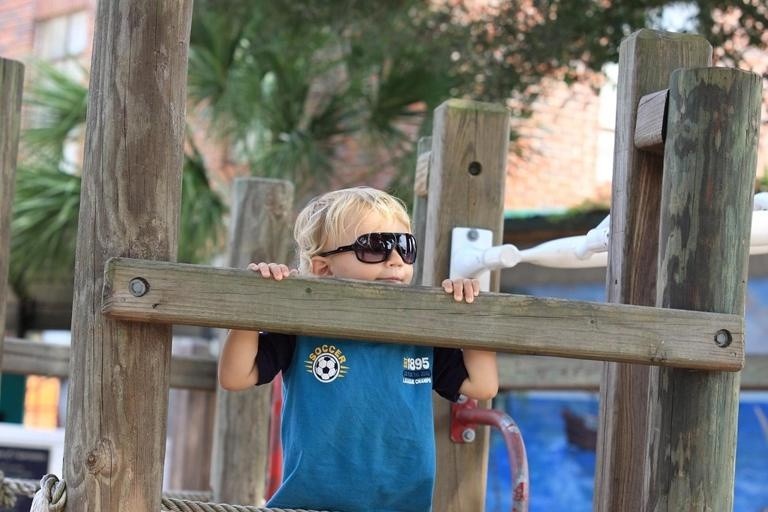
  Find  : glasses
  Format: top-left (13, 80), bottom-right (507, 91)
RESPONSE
top-left (318, 232), bottom-right (416, 266)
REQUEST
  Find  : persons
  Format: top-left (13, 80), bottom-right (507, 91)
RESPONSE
top-left (215, 183), bottom-right (502, 512)
